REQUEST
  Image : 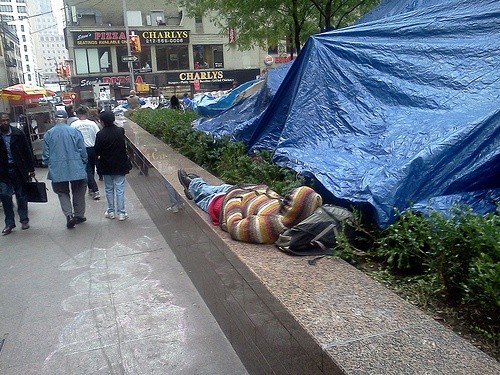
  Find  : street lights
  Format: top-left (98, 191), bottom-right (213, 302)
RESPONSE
top-left (46, 58), bottom-right (62, 96)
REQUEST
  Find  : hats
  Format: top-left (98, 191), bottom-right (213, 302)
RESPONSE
top-left (76, 107), bottom-right (87, 114)
top-left (55, 110), bottom-right (68, 118)
top-left (97, 110), bottom-right (115, 122)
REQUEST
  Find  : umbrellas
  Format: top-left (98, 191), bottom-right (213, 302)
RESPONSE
top-left (0, 83), bottom-right (55, 113)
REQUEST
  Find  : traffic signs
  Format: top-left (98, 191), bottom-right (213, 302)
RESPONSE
top-left (121, 56), bottom-right (139, 63)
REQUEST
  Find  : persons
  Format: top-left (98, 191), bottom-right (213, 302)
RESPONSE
top-left (144, 62), bottom-right (150, 68)
top-left (195, 61), bottom-right (200, 69)
top-left (70, 108), bottom-right (101, 200)
top-left (134, 62), bottom-right (140, 69)
top-left (182, 93), bottom-right (191, 111)
top-left (203, 61), bottom-right (209, 69)
top-left (0, 113), bottom-right (36, 234)
top-left (64, 105), bottom-right (79, 125)
top-left (95, 111), bottom-right (129, 221)
top-left (178, 168), bottom-right (323, 244)
top-left (87, 109), bottom-right (103, 181)
top-left (170, 92), bottom-right (180, 109)
top-left (41, 110), bottom-right (88, 228)
top-left (127, 90), bottom-right (143, 110)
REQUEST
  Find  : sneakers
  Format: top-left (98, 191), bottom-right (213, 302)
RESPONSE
top-left (104, 211), bottom-right (116, 219)
top-left (118, 211), bottom-right (128, 220)
top-left (87, 188), bottom-right (101, 199)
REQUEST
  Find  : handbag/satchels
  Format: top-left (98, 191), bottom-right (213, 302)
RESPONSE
top-left (275, 203), bottom-right (375, 265)
top-left (26, 175), bottom-right (48, 202)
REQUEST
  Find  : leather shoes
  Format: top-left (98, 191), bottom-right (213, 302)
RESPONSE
top-left (66, 215), bottom-right (76, 228)
top-left (178, 167), bottom-right (200, 201)
top-left (22, 222), bottom-right (29, 229)
top-left (74, 216), bottom-right (86, 224)
top-left (2, 224), bottom-right (17, 233)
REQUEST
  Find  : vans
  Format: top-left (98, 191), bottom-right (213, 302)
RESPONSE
top-left (39, 93), bottom-right (74, 119)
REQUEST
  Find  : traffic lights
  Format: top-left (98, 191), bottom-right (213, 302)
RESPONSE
top-left (131, 36), bottom-right (141, 53)
top-left (57, 67), bottom-right (62, 77)
